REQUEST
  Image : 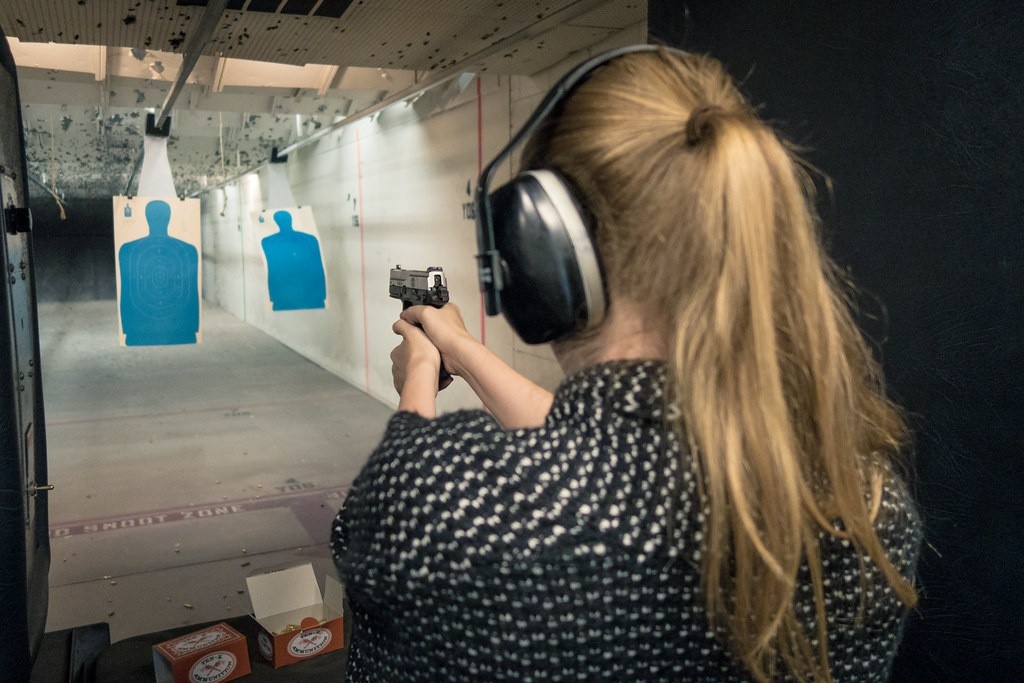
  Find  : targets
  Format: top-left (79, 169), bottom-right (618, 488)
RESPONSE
top-left (113, 194), bottom-right (203, 345)
top-left (250, 206), bottom-right (331, 309)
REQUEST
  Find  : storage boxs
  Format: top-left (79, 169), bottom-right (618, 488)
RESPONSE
top-left (242, 561), bottom-right (345, 669)
top-left (152, 622), bottom-right (251, 683)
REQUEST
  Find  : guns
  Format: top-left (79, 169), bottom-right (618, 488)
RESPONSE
top-left (388, 263), bottom-right (455, 391)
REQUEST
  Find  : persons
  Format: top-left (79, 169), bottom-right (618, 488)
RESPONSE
top-left (330, 40), bottom-right (924, 683)
